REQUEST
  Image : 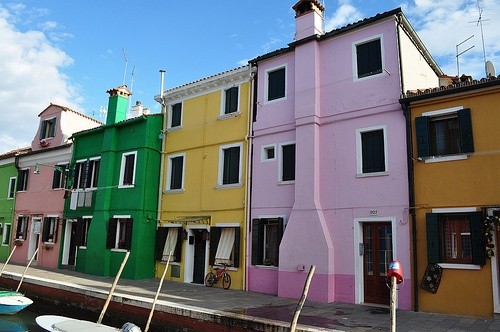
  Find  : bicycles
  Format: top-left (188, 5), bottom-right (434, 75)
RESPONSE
top-left (205, 262), bottom-right (234, 290)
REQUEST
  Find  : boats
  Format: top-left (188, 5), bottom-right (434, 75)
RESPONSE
top-left (36, 314), bottom-right (140, 332)
top-left (0, 288), bottom-right (33, 314)
